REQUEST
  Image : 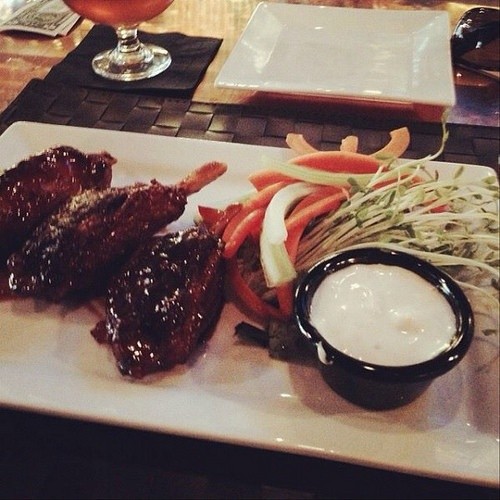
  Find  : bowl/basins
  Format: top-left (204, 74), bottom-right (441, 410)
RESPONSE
top-left (294, 248), bottom-right (475, 410)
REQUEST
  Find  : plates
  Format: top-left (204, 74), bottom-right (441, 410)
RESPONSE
top-left (0, 122), bottom-right (500, 484)
top-left (215, 2), bottom-right (455, 107)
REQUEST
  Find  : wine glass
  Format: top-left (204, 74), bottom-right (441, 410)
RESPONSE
top-left (63, 0), bottom-right (175, 81)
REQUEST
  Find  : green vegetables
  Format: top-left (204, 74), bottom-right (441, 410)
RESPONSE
top-left (246, 105), bottom-right (500, 336)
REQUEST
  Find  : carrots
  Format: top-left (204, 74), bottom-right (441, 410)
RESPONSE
top-left (197, 150), bottom-right (454, 319)
